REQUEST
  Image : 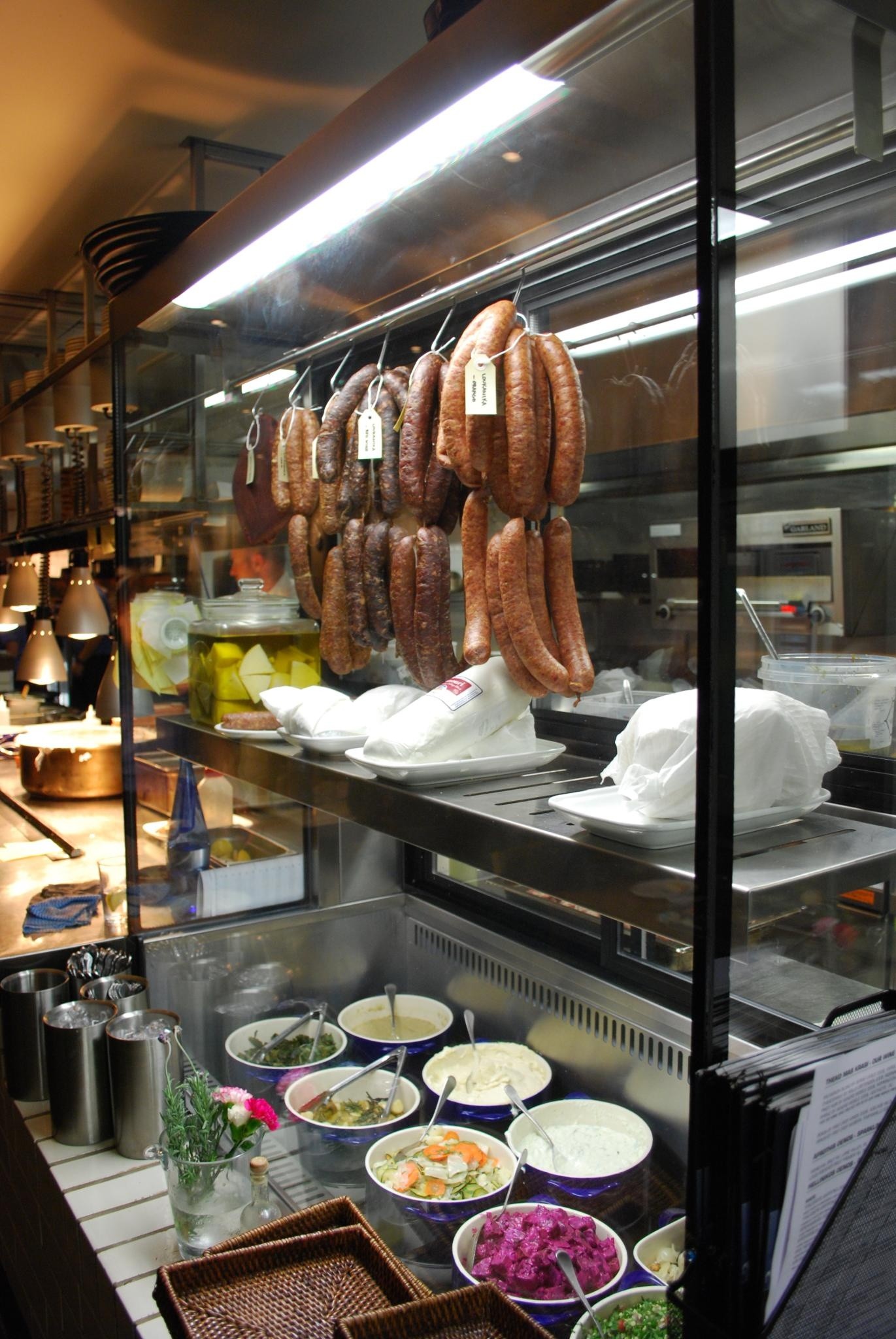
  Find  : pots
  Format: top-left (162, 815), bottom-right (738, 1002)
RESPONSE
top-left (223, 992), bottom-right (686, 1339)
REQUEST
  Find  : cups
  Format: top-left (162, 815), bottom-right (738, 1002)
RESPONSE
top-left (98, 858), bottom-right (128, 926)
top-left (0, 964), bottom-right (184, 1157)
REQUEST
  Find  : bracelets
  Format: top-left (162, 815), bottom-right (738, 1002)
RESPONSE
top-left (76, 658), bottom-right (84, 664)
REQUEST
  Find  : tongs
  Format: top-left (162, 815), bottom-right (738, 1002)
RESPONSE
top-left (246, 1000), bottom-right (334, 1069)
top-left (299, 1045), bottom-right (407, 1129)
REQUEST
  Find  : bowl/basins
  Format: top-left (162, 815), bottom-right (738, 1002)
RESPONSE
top-left (758, 652), bottom-right (895, 753)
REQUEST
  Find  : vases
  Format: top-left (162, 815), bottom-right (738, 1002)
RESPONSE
top-left (143, 1128), bottom-right (266, 1262)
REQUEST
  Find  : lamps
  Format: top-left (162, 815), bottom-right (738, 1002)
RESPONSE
top-left (0, 549), bottom-right (109, 685)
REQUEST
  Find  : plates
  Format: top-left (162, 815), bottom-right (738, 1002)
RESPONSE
top-left (143, 813), bottom-right (254, 842)
top-left (546, 784), bottom-right (833, 849)
top-left (345, 736), bottom-right (568, 786)
top-left (212, 719), bottom-right (284, 742)
top-left (273, 724), bottom-right (367, 758)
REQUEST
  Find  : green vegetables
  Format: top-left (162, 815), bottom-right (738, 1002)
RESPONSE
top-left (159, 1070), bottom-right (233, 1238)
top-left (313, 1091), bottom-right (390, 1128)
top-left (579, 1294), bottom-right (680, 1339)
top-left (238, 1032), bottom-right (337, 1067)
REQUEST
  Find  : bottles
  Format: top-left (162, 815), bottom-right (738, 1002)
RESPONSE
top-left (188, 579), bottom-right (322, 725)
top-left (194, 765), bottom-right (235, 831)
top-left (165, 757), bottom-right (211, 924)
top-left (83, 705), bottom-right (102, 724)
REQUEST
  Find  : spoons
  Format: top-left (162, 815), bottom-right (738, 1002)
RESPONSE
top-left (467, 1148), bottom-right (529, 1271)
top-left (463, 1008), bottom-right (482, 1093)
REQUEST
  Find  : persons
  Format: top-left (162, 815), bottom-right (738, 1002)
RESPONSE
top-left (60, 549), bottom-right (114, 708)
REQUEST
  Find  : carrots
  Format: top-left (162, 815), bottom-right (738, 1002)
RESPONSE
top-left (393, 1130), bottom-right (499, 1197)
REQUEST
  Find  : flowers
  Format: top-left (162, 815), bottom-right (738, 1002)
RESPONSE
top-left (134, 1023), bottom-right (281, 1209)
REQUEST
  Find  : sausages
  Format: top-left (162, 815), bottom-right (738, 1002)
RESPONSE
top-left (269, 300), bottom-right (596, 699)
